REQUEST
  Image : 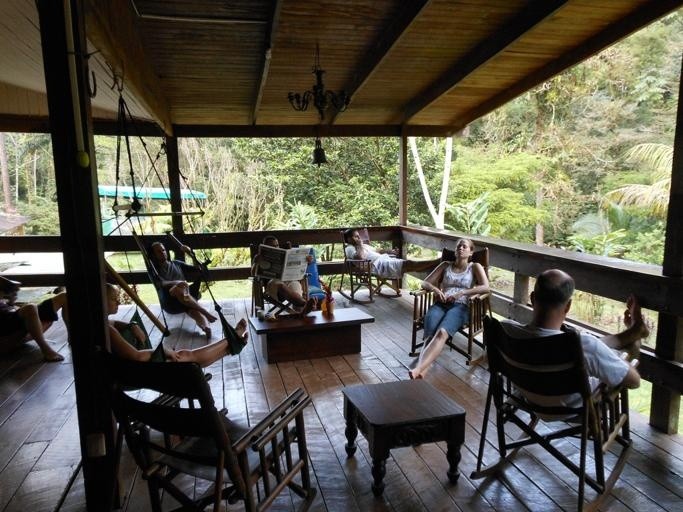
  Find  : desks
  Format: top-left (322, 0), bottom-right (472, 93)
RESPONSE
top-left (343, 380), bottom-right (467, 497)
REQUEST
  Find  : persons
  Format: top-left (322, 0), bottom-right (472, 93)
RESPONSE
top-left (252, 235), bottom-right (319, 319)
top-left (104, 281), bottom-right (248, 366)
top-left (493, 268), bottom-right (650, 422)
top-left (149, 240), bottom-right (217, 341)
top-left (407, 236), bottom-right (492, 381)
top-left (343, 228), bottom-right (443, 280)
top-left (1, 273), bottom-right (71, 364)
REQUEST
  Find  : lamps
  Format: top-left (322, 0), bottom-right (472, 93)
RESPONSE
top-left (287, 43), bottom-right (352, 121)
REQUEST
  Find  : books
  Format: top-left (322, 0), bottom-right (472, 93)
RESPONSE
top-left (255, 243), bottom-right (311, 282)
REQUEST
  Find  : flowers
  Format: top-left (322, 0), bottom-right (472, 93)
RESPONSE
top-left (317, 273), bottom-right (334, 302)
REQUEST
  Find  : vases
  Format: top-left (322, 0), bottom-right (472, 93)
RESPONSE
top-left (321, 299), bottom-right (335, 315)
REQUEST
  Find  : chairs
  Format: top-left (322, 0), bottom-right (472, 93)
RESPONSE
top-left (468, 317), bottom-right (632, 512)
top-left (91, 345), bottom-right (317, 510)
top-left (336, 230), bottom-right (401, 304)
top-left (248, 243), bottom-right (314, 314)
top-left (0, 301), bottom-right (57, 345)
top-left (408, 246), bottom-right (491, 365)
